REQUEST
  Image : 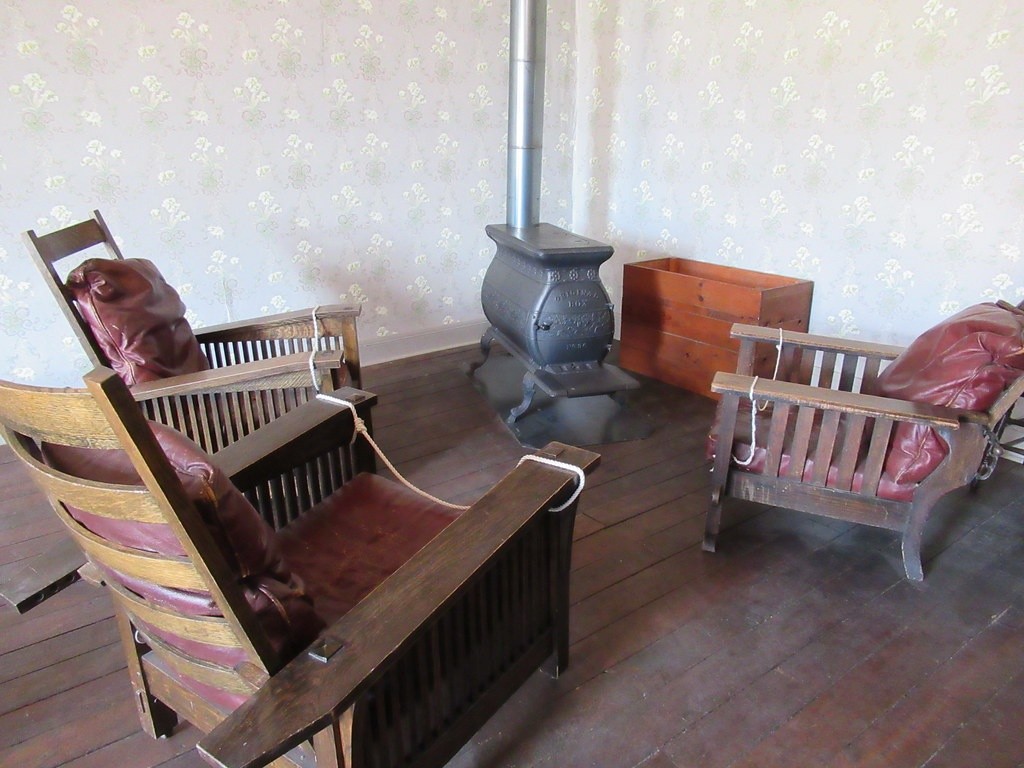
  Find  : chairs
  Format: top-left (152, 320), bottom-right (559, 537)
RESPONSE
top-left (701, 300), bottom-right (1024, 581)
top-left (19, 210), bottom-right (363, 455)
top-left (0, 366), bottom-right (602, 768)
